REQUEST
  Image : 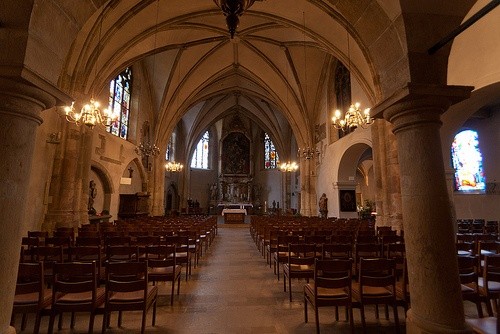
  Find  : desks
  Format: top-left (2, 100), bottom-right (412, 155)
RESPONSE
top-left (221, 208), bottom-right (248, 223)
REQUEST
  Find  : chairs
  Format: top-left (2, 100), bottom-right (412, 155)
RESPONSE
top-left (249, 214), bottom-right (411, 334)
top-left (9, 214), bottom-right (217, 334)
top-left (456, 217), bottom-right (500, 334)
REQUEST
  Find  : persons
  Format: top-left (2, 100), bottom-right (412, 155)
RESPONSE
top-left (319, 193), bottom-right (328, 219)
top-left (88, 180), bottom-right (97, 215)
top-left (194, 200), bottom-right (200, 214)
top-left (356, 203), bottom-right (363, 219)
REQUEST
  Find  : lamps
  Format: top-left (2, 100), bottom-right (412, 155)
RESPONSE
top-left (164, 50), bottom-right (185, 173)
top-left (64, 0), bottom-right (115, 129)
top-left (134, 0), bottom-right (161, 158)
top-left (296, 11), bottom-right (321, 160)
top-left (330, 24), bottom-right (373, 130)
top-left (278, 63), bottom-right (299, 173)
